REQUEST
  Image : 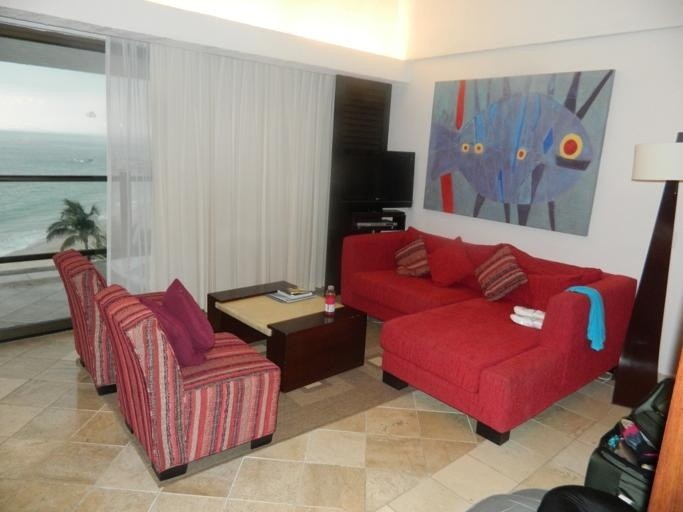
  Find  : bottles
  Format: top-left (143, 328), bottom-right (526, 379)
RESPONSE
top-left (325, 286), bottom-right (336, 318)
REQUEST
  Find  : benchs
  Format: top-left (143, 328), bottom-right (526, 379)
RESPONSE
top-left (340, 230), bottom-right (637, 446)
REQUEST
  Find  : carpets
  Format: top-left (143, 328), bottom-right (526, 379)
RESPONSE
top-left (75, 305), bottom-right (417, 488)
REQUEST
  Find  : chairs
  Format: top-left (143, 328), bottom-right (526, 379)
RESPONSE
top-left (94, 282), bottom-right (282, 481)
top-left (52, 248), bottom-right (166, 396)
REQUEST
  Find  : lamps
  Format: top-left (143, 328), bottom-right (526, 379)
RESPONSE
top-left (612, 132), bottom-right (683, 410)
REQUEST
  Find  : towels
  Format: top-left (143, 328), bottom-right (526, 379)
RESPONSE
top-left (510, 305), bottom-right (545, 330)
top-left (566, 285), bottom-right (606, 352)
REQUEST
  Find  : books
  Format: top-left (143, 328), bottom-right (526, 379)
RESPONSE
top-left (268, 287), bottom-right (317, 304)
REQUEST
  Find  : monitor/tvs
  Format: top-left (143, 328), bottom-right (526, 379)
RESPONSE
top-left (337, 148), bottom-right (415, 215)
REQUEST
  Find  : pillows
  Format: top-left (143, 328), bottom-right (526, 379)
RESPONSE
top-left (394, 225), bottom-right (603, 311)
top-left (137, 297), bottom-right (206, 367)
top-left (162, 276), bottom-right (215, 350)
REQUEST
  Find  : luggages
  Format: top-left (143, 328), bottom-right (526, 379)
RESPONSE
top-left (584, 375), bottom-right (678, 512)
